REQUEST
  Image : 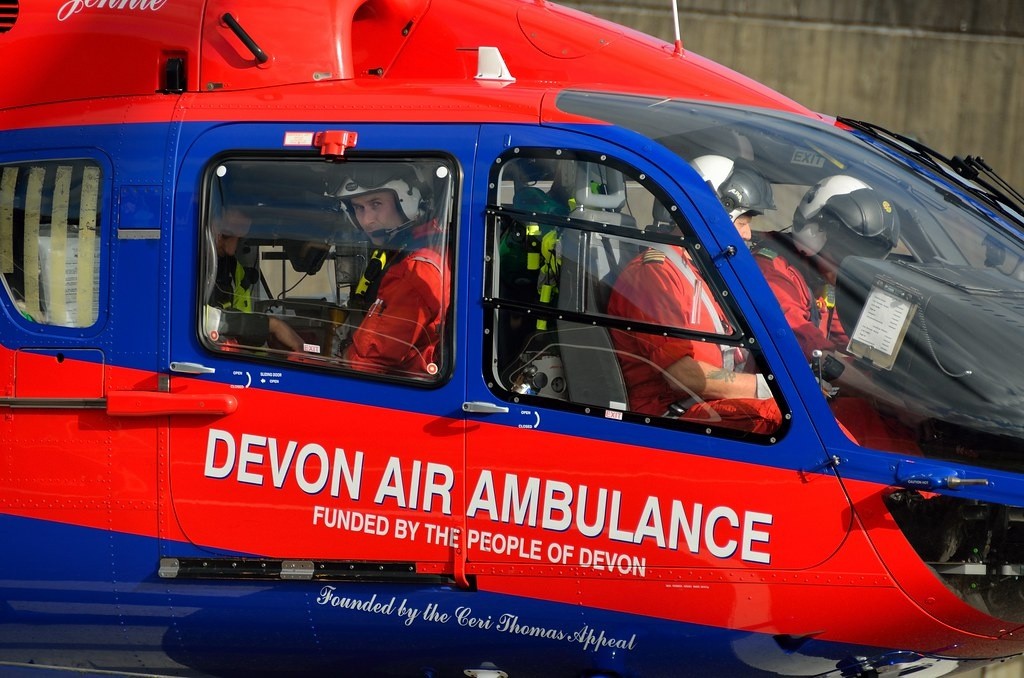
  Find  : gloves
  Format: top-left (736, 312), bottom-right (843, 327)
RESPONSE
top-left (756, 370), bottom-right (839, 407)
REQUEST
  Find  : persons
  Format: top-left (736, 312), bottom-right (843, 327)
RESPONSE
top-left (608, 148), bottom-right (859, 446)
top-left (331, 163), bottom-right (451, 376)
top-left (752, 174), bottom-right (925, 459)
top-left (203, 186), bottom-right (309, 354)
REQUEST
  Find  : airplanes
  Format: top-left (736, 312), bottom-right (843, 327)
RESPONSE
top-left (0, 0), bottom-right (1024, 678)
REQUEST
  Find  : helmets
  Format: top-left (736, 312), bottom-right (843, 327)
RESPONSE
top-left (652, 154), bottom-right (778, 227)
top-left (794, 174), bottom-right (899, 257)
top-left (334, 159), bottom-right (446, 235)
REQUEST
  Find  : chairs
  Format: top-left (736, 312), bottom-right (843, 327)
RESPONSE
top-left (555, 162), bottom-right (648, 412)
